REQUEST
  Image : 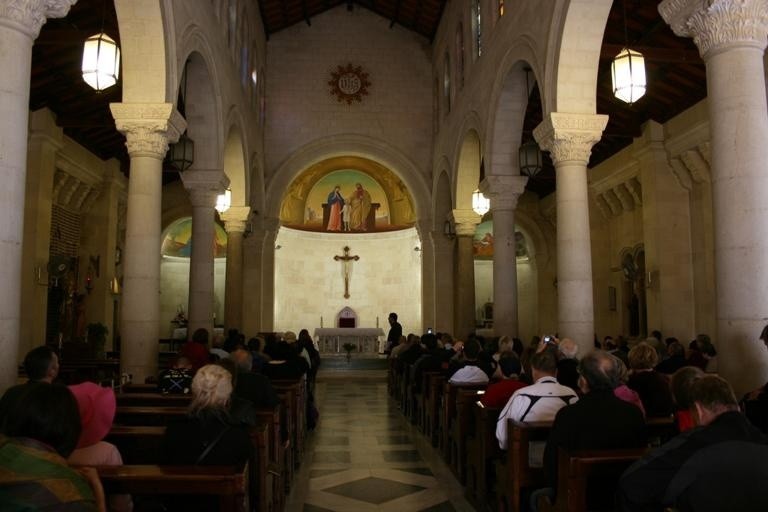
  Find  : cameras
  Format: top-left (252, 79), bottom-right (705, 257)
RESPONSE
top-left (544, 336), bottom-right (552, 343)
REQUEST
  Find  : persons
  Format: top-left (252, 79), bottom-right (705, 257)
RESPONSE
top-left (398, 333), bottom-right (421, 371)
top-left (495, 352), bottom-right (580, 465)
top-left (647, 366), bottom-right (710, 445)
top-left (688, 334), bottom-right (711, 353)
top-left (626, 342), bottom-right (676, 417)
top-left (327, 185), bottom-right (344, 232)
top-left (446, 341), bottom-right (488, 382)
top-left (391, 336), bottom-right (406, 361)
top-left (223, 328), bottom-right (312, 364)
top-left (339, 198), bottom-right (352, 232)
top-left (645, 337), bottom-right (662, 364)
top-left (738, 325), bottom-right (768, 436)
top-left (521, 346), bottom-right (538, 385)
top-left (384, 313), bottom-right (402, 345)
top-left (160, 363), bottom-right (252, 467)
top-left (557, 337), bottom-right (584, 385)
top-left (611, 351), bottom-right (647, 422)
top-left (663, 337), bottom-right (679, 360)
top-left (480, 352), bottom-right (531, 407)
top-left (493, 335), bottom-right (526, 379)
top-left (235, 349), bottom-right (279, 409)
top-left (23, 346), bottom-right (60, 387)
top-left (655, 342), bottom-right (689, 374)
top-left (350, 184), bottom-right (372, 232)
top-left (411, 334), bottom-right (447, 374)
top-left (156, 358), bottom-right (193, 394)
top-left (689, 349), bottom-right (716, 372)
top-left (619, 373), bottom-right (768, 512)
top-left (650, 331), bottom-right (666, 353)
top-left (608, 336), bottom-right (632, 370)
top-left (65, 382), bottom-right (124, 466)
top-left (477, 337), bottom-right (488, 358)
top-left (481, 232), bottom-right (495, 245)
top-left (1, 383), bottom-right (100, 512)
top-left (543, 350), bottom-right (650, 505)
top-left (169, 328), bottom-right (209, 378)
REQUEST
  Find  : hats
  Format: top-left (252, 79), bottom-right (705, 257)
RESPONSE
top-left (68, 382), bottom-right (117, 450)
top-left (0, 382), bottom-right (82, 460)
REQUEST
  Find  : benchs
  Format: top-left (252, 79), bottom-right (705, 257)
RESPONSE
top-left (115, 405), bottom-right (285, 512)
top-left (124, 383), bottom-right (303, 457)
top-left (506, 417), bottom-right (556, 512)
top-left (107, 423), bottom-right (272, 512)
top-left (412, 371), bottom-right (441, 435)
top-left (424, 376), bottom-right (448, 448)
top-left (464, 402), bottom-right (505, 512)
top-left (70, 460), bottom-right (250, 512)
top-left (438, 380), bottom-right (492, 460)
top-left (556, 447), bottom-right (648, 512)
top-left (115, 391), bottom-right (292, 483)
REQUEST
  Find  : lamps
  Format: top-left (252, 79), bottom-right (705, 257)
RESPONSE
top-left (215, 187), bottom-right (232, 215)
top-left (169, 59), bottom-right (195, 175)
top-left (81, 0), bottom-right (121, 96)
top-left (519, 67), bottom-right (543, 178)
top-left (471, 188), bottom-right (491, 218)
top-left (610, 0), bottom-right (647, 107)
